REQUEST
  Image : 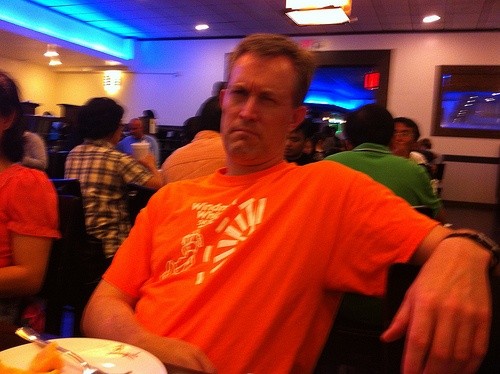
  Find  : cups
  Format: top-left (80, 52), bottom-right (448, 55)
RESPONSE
top-left (130, 142), bottom-right (151, 161)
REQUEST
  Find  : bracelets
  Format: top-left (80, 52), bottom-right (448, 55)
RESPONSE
top-left (443, 230), bottom-right (499, 256)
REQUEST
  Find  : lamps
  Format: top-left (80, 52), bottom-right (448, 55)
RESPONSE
top-left (282, 0), bottom-right (352, 27)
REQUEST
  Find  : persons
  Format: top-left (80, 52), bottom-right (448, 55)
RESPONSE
top-left (22, 127), bottom-right (48, 170)
top-left (0, 73), bottom-right (63, 344)
top-left (161, 81), bottom-right (446, 218)
top-left (80, 33), bottom-right (500, 374)
top-left (41, 96), bottom-right (163, 264)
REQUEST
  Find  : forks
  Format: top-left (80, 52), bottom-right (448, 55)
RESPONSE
top-left (15, 326), bottom-right (132, 374)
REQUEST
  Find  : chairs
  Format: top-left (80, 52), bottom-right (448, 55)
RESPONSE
top-left (23, 99), bottom-right (157, 342)
top-left (316, 263), bottom-right (500, 374)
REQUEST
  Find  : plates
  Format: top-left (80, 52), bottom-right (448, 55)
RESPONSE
top-left (0, 337), bottom-right (169, 374)
